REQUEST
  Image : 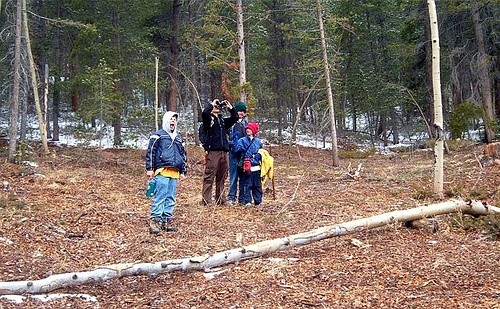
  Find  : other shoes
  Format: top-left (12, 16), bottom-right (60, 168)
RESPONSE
top-left (205, 202), bottom-right (216, 209)
top-left (238, 201), bottom-right (245, 207)
top-left (161, 221), bottom-right (178, 232)
top-left (226, 200), bottom-right (235, 206)
top-left (246, 202), bottom-right (252, 207)
top-left (147, 220), bottom-right (162, 233)
top-left (218, 202), bottom-right (233, 207)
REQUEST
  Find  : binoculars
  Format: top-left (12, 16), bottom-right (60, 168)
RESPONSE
top-left (215, 100), bottom-right (229, 109)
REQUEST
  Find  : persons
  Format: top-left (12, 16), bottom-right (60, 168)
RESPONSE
top-left (145, 111), bottom-right (187, 233)
top-left (201, 99), bottom-right (240, 206)
top-left (225, 102), bottom-right (264, 207)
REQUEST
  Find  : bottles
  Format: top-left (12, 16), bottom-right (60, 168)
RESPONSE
top-left (146, 178), bottom-right (158, 197)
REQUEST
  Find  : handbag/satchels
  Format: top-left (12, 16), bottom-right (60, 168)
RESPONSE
top-left (237, 157), bottom-right (252, 172)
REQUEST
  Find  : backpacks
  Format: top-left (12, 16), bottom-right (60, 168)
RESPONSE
top-left (199, 116), bottom-right (227, 146)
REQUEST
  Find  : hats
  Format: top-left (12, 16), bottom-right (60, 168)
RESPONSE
top-left (244, 121), bottom-right (259, 136)
top-left (234, 101), bottom-right (248, 113)
